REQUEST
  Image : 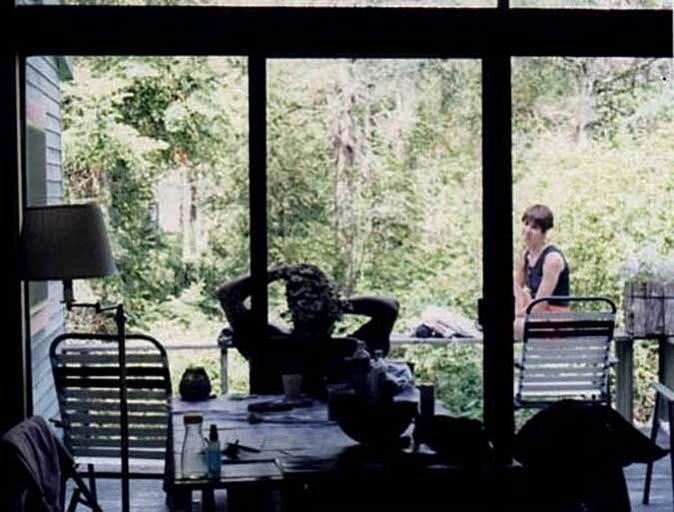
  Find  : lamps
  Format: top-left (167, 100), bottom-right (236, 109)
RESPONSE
top-left (17, 203), bottom-right (130, 336)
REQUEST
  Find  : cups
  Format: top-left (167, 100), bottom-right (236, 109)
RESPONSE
top-left (281, 373), bottom-right (301, 399)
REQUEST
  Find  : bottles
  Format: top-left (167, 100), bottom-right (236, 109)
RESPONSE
top-left (351, 340), bottom-right (369, 360)
top-left (180, 413), bottom-right (208, 480)
top-left (206, 423), bottom-right (221, 474)
top-left (370, 348), bottom-right (386, 391)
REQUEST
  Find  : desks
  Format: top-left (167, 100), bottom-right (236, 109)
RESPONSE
top-left (166, 389), bottom-right (380, 511)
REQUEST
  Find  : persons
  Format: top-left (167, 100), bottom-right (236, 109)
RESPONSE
top-left (216, 265), bottom-right (398, 397)
top-left (514, 204), bottom-right (570, 340)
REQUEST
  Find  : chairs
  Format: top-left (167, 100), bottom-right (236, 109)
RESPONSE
top-left (513, 294), bottom-right (621, 425)
top-left (40, 333), bottom-right (179, 512)
top-left (1, 415), bottom-right (103, 512)
